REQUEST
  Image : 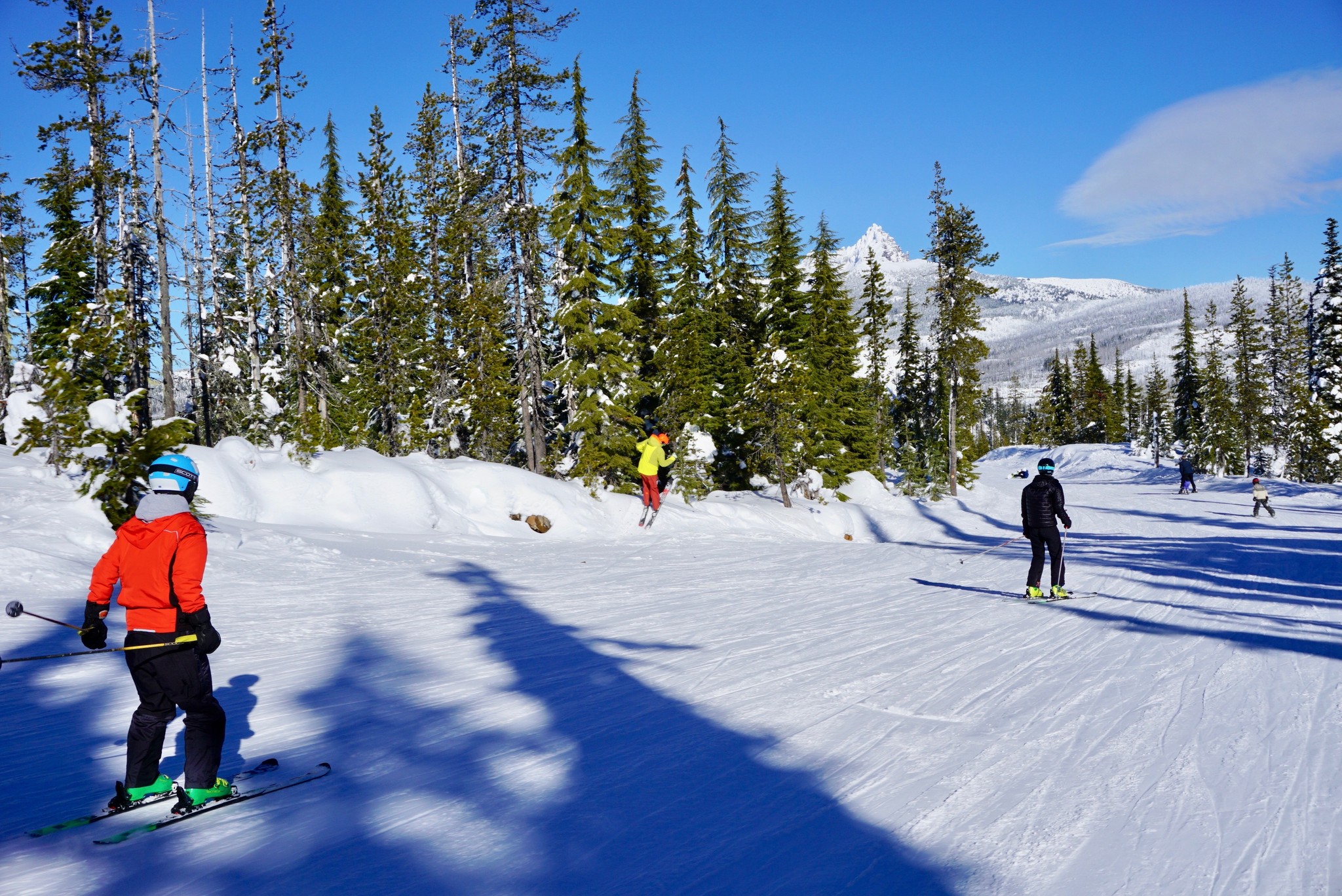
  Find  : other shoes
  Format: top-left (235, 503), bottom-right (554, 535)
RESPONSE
top-left (1017, 471), bottom-right (1019, 474)
top-left (1012, 474), bottom-right (1014, 478)
top-left (1184, 489), bottom-right (1189, 494)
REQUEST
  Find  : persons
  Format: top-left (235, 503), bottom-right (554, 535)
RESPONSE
top-left (1251, 478), bottom-right (1275, 517)
top-left (635, 433), bottom-right (678, 511)
top-left (1020, 458), bottom-right (1072, 599)
top-left (1181, 477), bottom-right (1191, 494)
top-left (1178, 456), bottom-right (1198, 494)
top-left (1012, 467), bottom-right (1029, 478)
top-left (81, 454), bottom-right (230, 808)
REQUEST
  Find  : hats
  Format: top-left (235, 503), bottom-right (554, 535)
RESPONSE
top-left (1181, 455), bottom-right (1186, 459)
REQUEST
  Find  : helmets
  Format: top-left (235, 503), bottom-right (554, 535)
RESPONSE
top-left (149, 454), bottom-right (199, 492)
top-left (1038, 458), bottom-right (1054, 475)
top-left (1252, 478), bottom-right (1259, 483)
top-left (658, 433), bottom-right (669, 445)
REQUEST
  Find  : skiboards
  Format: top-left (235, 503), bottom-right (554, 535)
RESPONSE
top-left (1171, 492), bottom-right (1199, 494)
top-left (639, 480), bottom-right (673, 528)
top-left (1249, 514), bottom-right (1279, 519)
top-left (22, 758), bottom-right (331, 845)
top-left (1002, 592), bottom-right (1098, 604)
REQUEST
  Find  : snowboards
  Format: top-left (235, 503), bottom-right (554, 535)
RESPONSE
top-left (1007, 468), bottom-right (1026, 479)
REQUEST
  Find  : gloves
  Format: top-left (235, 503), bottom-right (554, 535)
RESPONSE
top-left (1061, 517), bottom-right (1071, 529)
top-left (1253, 497), bottom-right (1256, 501)
top-left (185, 603), bottom-right (221, 654)
top-left (1266, 497), bottom-right (1268, 501)
top-left (672, 453), bottom-right (678, 459)
top-left (1023, 529), bottom-right (1030, 539)
top-left (81, 600), bottom-right (110, 650)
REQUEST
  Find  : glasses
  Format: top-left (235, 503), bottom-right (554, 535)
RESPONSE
top-left (663, 444), bottom-right (666, 446)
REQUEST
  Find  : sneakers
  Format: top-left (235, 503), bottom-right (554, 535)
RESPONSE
top-left (1179, 489), bottom-right (1184, 493)
top-left (1271, 513), bottom-right (1275, 516)
top-left (186, 778), bottom-right (231, 805)
top-left (1029, 586), bottom-right (1043, 597)
top-left (1254, 513), bottom-right (1258, 516)
top-left (1192, 488), bottom-right (1197, 493)
top-left (1052, 585), bottom-right (1066, 596)
top-left (127, 774), bottom-right (173, 801)
